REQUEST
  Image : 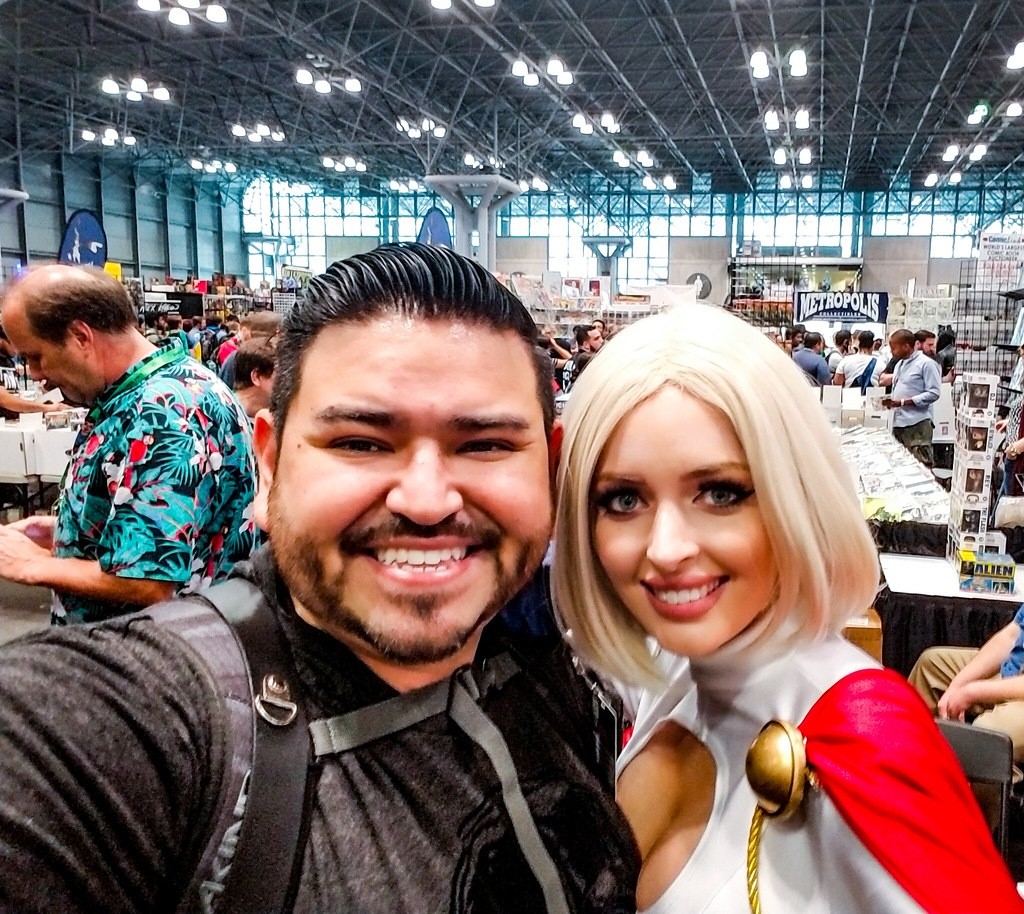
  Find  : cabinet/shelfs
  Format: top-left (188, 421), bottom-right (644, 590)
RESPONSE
top-left (990, 266), bottom-right (1024, 420)
top-left (488, 272), bottom-right (663, 352)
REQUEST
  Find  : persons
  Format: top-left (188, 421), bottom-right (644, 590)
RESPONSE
top-left (541, 301), bottom-right (1024, 913)
top-left (1, 239), bottom-right (641, 914)
top-left (908, 599), bottom-right (1024, 790)
top-left (1, 267), bottom-right (1024, 495)
top-left (1, 260), bottom-right (260, 626)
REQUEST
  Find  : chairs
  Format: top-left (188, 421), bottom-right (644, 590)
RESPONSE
top-left (933, 716), bottom-right (1012, 864)
top-left (0, 425), bottom-right (43, 519)
top-left (40, 429), bottom-right (81, 517)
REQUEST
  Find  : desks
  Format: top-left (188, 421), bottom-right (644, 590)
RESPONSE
top-left (878, 552), bottom-right (1024, 682)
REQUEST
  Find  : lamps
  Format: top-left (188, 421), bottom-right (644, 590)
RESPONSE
top-left (79, 0), bottom-right (1024, 196)
